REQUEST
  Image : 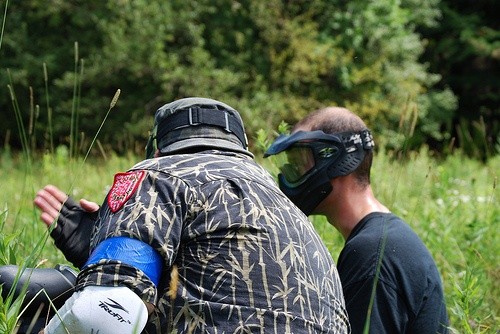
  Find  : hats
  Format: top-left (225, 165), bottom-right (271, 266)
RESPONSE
top-left (143, 95), bottom-right (256, 160)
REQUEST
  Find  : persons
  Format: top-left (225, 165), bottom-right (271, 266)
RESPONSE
top-left (260, 103), bottom-right (451, 334)
top-left (31, 98), bottom-right (352, 334)
